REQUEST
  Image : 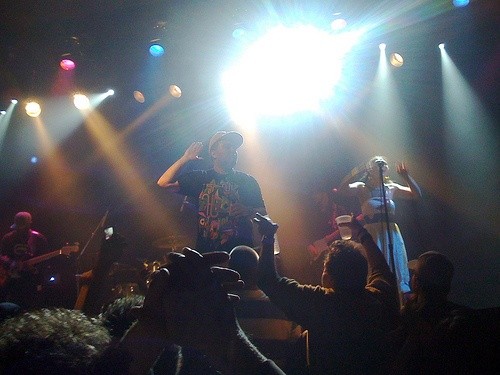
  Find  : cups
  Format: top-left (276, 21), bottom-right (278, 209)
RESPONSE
top-left (335, 215), bottom-right (353, 240)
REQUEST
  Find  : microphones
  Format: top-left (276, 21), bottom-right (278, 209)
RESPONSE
top-left (379, 162), bottom-right (384, 167)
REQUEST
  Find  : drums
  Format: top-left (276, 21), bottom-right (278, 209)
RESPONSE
top-left (111, 282), bottom-right (139, 299)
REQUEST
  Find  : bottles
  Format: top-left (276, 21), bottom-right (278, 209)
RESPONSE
top-left (264, 215), bottom-right (280, 255)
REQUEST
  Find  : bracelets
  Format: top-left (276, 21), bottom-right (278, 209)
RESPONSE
top-left (358, 232), bottom-right (369, 242)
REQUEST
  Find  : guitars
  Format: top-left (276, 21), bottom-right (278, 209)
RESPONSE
top-left (0, 244), bottom-right (80, 284)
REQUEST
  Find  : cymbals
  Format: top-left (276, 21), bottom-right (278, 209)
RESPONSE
top-left (152, 235), bottom-right (191, 249)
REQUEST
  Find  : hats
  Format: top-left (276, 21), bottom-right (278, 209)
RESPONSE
top-left (407, 251), bottom-right (454, 282)
top-left (10, 212), bottom-right (32, 230)
top-left (209, 131), bottom-right (243, 157)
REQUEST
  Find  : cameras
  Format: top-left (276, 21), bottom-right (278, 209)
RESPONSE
top-left (103, 226), bottom-right (118, 241)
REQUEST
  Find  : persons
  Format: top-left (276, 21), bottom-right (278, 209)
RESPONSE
top-left (341, 156), bottom-right (421, 294)
top-left (0, 212), bottom-right (500, 375)
top-left (158, 130), bottom-right (266, 252)
